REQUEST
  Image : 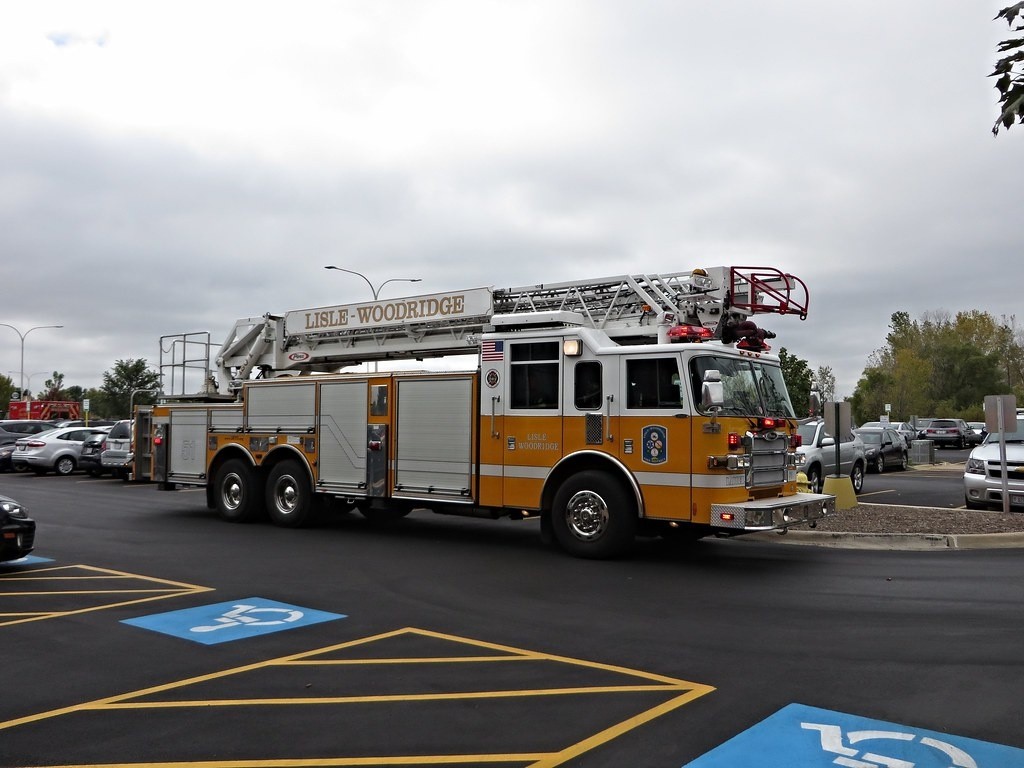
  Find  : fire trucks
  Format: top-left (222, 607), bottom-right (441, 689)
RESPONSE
top-left (9, 401), bottom-right (79, 421)
top-left (134, 265), bottom-right (837, 556)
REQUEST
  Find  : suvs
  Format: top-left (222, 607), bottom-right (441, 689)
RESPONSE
top-left (0, 420), bottom-right (60, 472)
top-left (79, 431), bottom-right (110, 475)
top-left (101, 419), bottom-right (134, 478)
top-left (909, 418), bottom-right (938, 438)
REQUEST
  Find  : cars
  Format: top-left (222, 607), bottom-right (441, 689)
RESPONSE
top-left (878, 422), bottom-right (917, 440)
top-left (923, 419), bottom-right (975, 449)
top-left (794, 420), bottom-right (867, 495)
top-left (964, 416), bottom-right (1024, 509)
top-left (11, 427), bottom-right (110, 475)
top-left (860, 421), bottom-right (880, 429)
top-left (0, 495), bottom-right (36, 560)
top-left (854, 427), bottom-right (909, 474)
top-left (967, 423), bottom-right (986, 437)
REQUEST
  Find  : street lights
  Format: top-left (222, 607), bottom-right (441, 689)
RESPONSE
top-left (324, 265), bottom-right (422, 372)
top-left (8, 371), bottom-right (48, 396)
top-left (0, 323), bottom-right (64, 399)
